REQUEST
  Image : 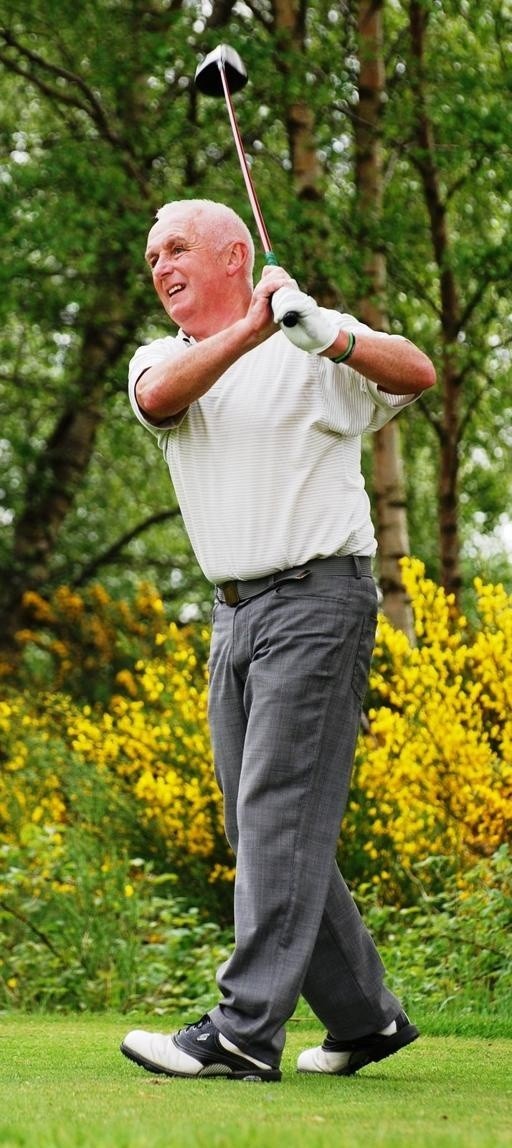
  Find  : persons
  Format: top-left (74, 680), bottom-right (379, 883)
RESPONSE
top-left (119, 199), bottom-right (438, 1079)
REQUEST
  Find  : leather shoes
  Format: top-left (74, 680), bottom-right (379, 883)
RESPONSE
top-left (298, 1008), bottom-right (418, 1074)
top-left (121, 1016), bottom-right (281, 1083)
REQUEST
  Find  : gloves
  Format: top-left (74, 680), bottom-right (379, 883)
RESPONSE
top-left (272, 279), bottom-right (341, 356)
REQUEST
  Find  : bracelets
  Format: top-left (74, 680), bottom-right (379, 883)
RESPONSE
top-left (332, 334), bottom-right (356, 363)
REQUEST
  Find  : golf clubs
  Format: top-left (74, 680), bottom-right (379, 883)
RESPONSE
top-left (194, 46), bottom-right (301, 330)
